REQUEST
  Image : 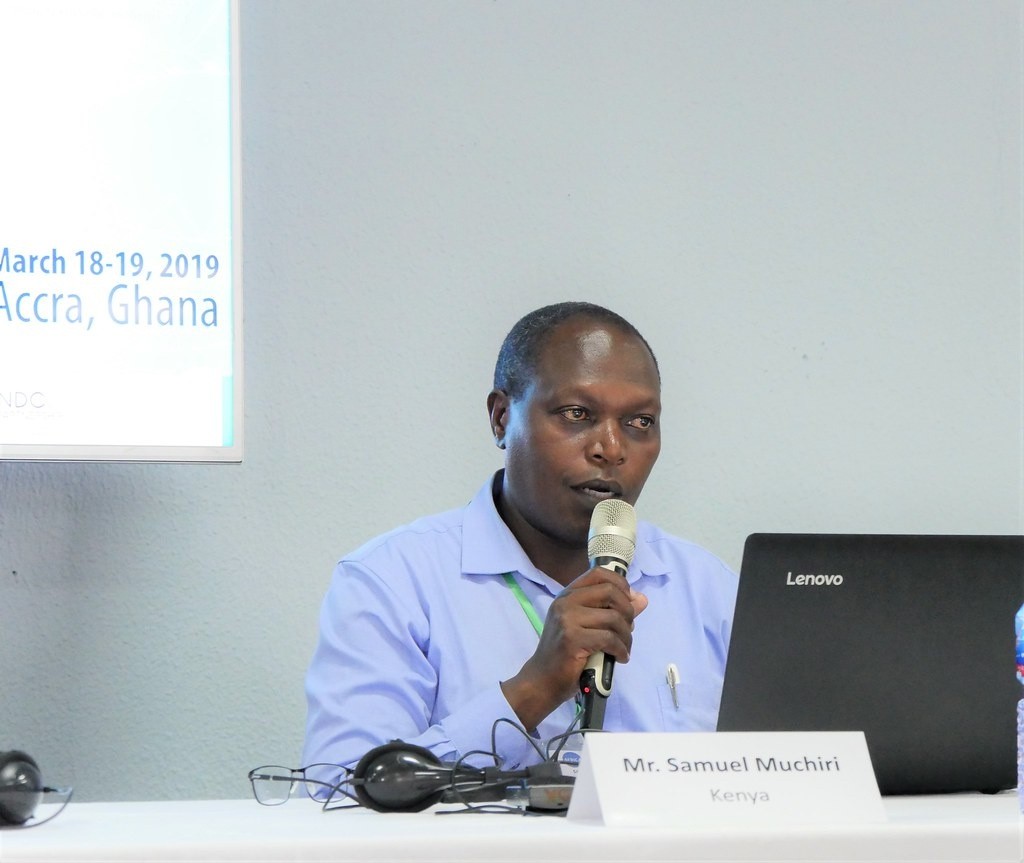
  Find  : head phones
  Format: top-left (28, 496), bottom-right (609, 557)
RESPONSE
top-left (351, 744), bottom-right (562, 813)
top-left (0, 750), bottom-right (73, 824)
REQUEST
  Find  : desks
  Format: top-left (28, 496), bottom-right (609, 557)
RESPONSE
top-left (0, 793), bottom-right (1024, 863)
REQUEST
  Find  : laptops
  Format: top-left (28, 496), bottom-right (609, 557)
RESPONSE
top-left (717, 532), bottom-right (1024, 796)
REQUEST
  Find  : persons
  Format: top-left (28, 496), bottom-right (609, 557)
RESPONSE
top-left (301, 302), bottom-right (739, 804)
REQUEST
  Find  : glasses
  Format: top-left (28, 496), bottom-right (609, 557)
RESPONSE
top-left (248, 758), bottom-right (355, 807)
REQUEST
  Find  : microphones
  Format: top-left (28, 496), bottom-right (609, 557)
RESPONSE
top-left (580, 499), bottom-right (637, 736)
